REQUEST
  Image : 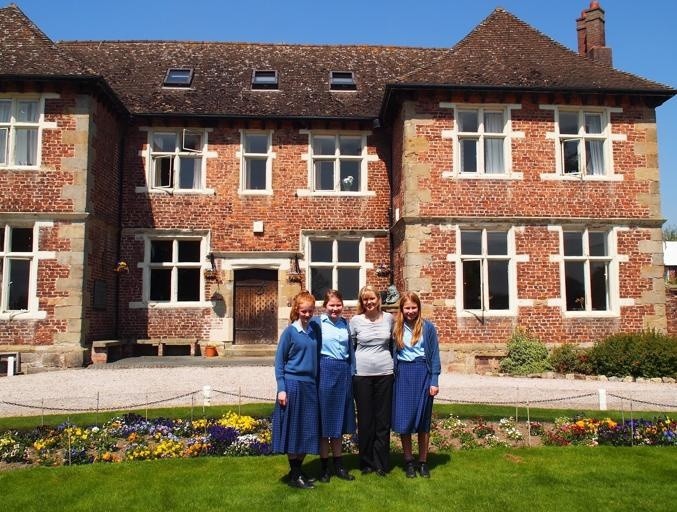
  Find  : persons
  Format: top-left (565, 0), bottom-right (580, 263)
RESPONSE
top-left (348, 285), bottom-right (395, 477)
top-left (271, 291), bottom-right (320, 488)
top-left (392, 291), bottom-right (442, 479)
top-left (310, 288), bottom-right (358, 482)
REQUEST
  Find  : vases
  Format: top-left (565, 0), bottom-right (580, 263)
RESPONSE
top-left (205, 346), bottom-right (217, 357)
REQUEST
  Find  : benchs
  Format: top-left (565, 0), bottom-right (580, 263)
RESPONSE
top-left (90, 338), bottom-right (198, 364)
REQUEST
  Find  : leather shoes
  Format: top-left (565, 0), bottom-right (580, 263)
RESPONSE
top-left (405, 462), bottom-right (416, 478)
top-left (320, 468), bottom-right (330, 483)
top-left (287, 471), bottom-right (317, 489)
top-left (362, 466), bottom-right (372, 475)
top-left (377, 469), bottom-right (384, 475)
top-left (418, 464), bottom-right (430, 478)
top-left (334, 467), bottom-right (354, 480)
